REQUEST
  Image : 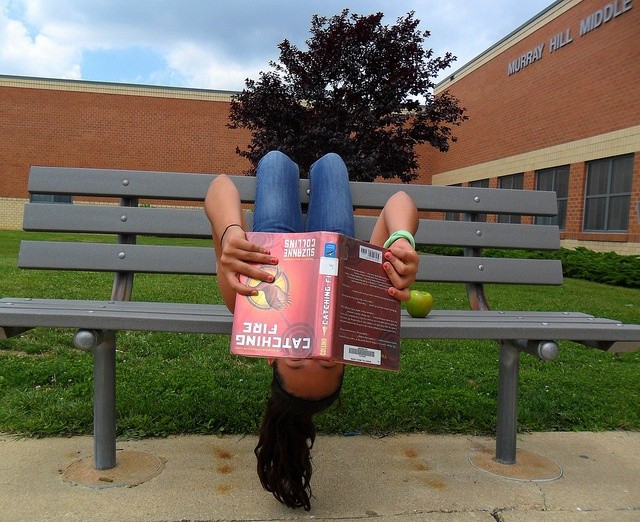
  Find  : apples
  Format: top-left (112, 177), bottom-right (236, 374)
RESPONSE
top-left (406, 289), bottom-right (433, 317)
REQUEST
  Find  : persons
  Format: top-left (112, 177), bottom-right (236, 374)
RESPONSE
top-left (203, 150), bottom-right (419, 513)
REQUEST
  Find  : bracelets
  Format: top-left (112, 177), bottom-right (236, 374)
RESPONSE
top-left (219, 224), bottom-right (245, 248)
top-left (382, 230), bottom-right (416, 250)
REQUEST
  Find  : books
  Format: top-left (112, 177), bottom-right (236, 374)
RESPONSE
top-left (229, 230), bottom-right (402, 373)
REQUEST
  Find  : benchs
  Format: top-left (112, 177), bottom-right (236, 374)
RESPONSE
top-left (0, 165), bottom-right (640, 469)
